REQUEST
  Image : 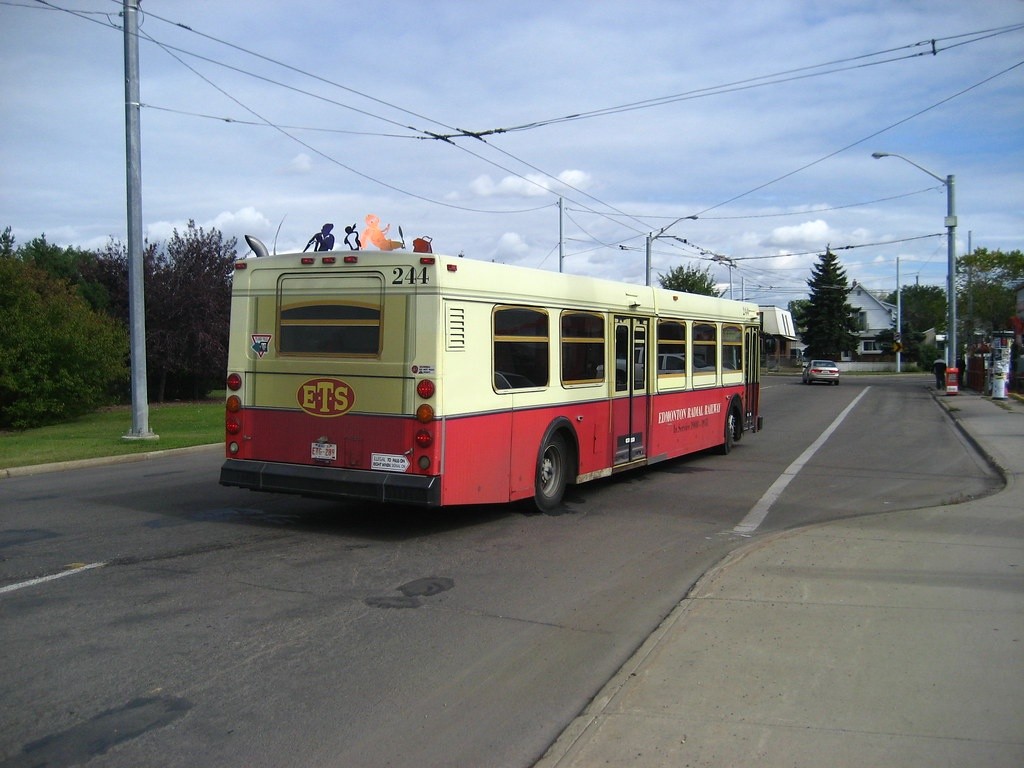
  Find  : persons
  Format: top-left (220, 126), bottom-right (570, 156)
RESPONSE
top-left (932, 354), bottom-right (946, 389)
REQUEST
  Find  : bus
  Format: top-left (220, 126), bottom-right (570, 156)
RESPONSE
top-left (216, 233), bottom-right (763, 514)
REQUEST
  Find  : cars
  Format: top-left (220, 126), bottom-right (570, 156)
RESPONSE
top-left (802, 360), bottom-right (840, 385)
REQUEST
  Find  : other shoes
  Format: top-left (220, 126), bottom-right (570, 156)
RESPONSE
top-left (943, 387), bottom-right (945, 389)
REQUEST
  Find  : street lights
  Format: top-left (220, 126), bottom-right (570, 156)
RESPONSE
top-left (646, 214), bottom-right (699, 288)
top-left (872, 151), bottom-right (959, 368)
